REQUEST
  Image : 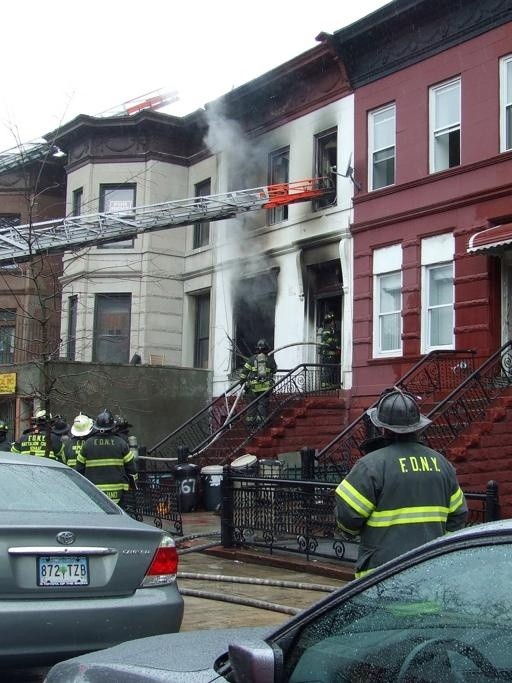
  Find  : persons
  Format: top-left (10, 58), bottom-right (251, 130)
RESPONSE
top-left (240, 338), bottom-right (277, 430)
top-left (61, 412), bottom-right (96, 470)
top-left (111, 412), bottom-right (140, 516)
top-left (332, 385), bottom-right (469, 619)
top-left (0, 419), bottom-right (15, 451)
top-left (9, 409), bottom-right (62, 463)
top-left (76, 408), bottom-right (138, 511)
top-left (51, 414), bottom-right (73, 444)
top-left (316, 311), bottom-right (340, 388)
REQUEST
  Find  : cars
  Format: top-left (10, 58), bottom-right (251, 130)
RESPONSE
top-left (43, 517), bottom-right (511, 681)
top-left (0, 450), bottom-right (185, 668)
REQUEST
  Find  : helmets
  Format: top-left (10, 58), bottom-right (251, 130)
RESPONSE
top-left (92, 412), bottom-right (118, 431)
top-left (368, 389), bottom-right (434, 433)
top-left (0, 420), bottom-right (9, 433)
top-left (255, 339), bottom-right (268, 348)
top-left (51, 420), bottom-right (70, 434)
top-left (70, 415), bottom-right (95, 436)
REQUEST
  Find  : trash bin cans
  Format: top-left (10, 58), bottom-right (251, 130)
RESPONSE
top-left (171, 453), bottom-right (281, 512)
